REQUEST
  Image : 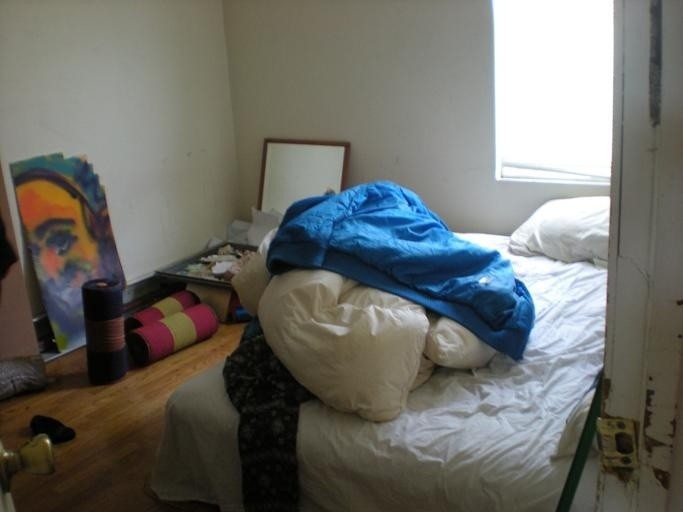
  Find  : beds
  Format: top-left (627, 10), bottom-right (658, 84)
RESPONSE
top-left (168, 223), bottom-right (605, 512)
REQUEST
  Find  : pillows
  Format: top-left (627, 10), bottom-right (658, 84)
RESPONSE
top-left (509, 188), bottom-right (612, 273)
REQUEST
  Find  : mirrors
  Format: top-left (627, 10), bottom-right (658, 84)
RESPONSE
top-left (255, 137), bottom-right (351, 222)
